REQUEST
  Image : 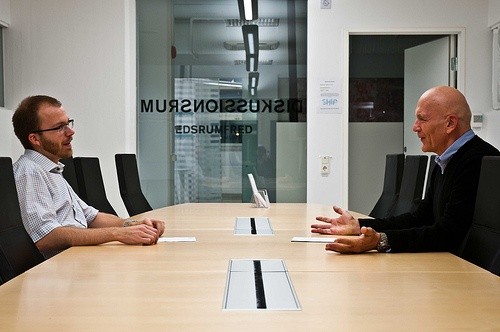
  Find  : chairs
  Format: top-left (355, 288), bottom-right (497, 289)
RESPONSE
top-left (0, 153), bottom-right (154, 288)
top-left (368, 153), bottom-right (500, 278)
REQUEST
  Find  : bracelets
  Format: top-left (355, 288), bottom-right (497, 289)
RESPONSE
top-left (124, 218), bottom-right (135, 227)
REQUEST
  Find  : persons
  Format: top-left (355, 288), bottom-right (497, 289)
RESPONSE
top-left (311, 85), bottom-right (500, 274)
top-left (184, 137), bottom-right (231, 190)
top-left (255, 146), bottom-right (275, 177)
top-left (13, 97), bottom-right (165, 245)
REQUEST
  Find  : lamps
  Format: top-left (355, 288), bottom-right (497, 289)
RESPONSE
top-left (237, 0), bottom-right (258, 21)
top-left (242, 25), bottom-right (260, 96)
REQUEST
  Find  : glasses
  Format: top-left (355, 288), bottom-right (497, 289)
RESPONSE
top-left (32, 119), bottom-right (74, 134)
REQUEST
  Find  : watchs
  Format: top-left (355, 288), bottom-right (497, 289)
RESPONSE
top-left (377, 233), bottom-right (387, 252)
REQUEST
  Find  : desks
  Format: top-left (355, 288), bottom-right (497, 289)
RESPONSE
top-left (1, 202), bottom-right (500, 331)
